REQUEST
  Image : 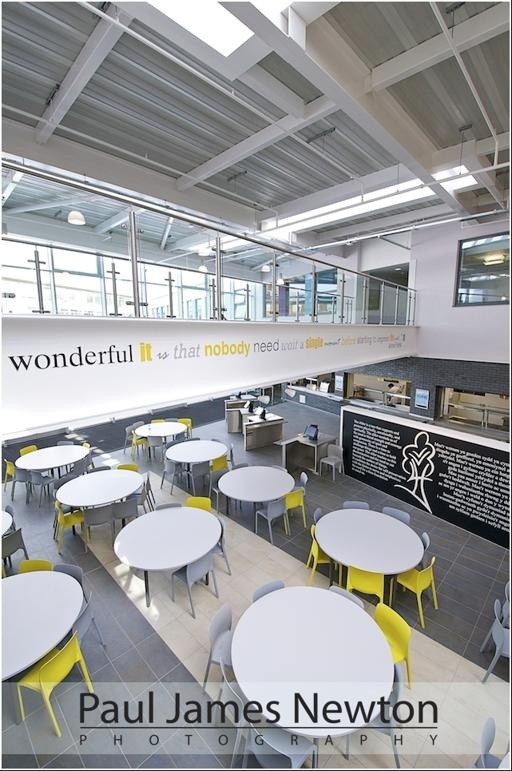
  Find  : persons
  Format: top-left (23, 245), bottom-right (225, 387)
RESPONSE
top-left (387, 383), bottom-right (402, 405)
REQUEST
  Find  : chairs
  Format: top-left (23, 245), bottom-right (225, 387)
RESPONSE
top-left (16, 631), bottom-right (96, 740)
top-left (368, 597), bottom-right (419, 693)
top-left (318, 442), bottom-right (347, 479)
top-left (244, 709), bottom-right (318, 770)
top-left (389, 557), bottom-right (439, 628)
top-left (346, 664), bottom-right (406, 769)
top-left (307, 498), bottom-right (430, 606)
top-left (214, 661), bottom-right (250, 766)
top-left (471, 717), bottom-right (502, 770)
top-left (502, 582), bottom-right (511, 628)
top-left (478, 593), bottom-right (511, 685)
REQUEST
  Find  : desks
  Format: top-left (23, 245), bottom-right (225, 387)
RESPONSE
top-left (3, 416), bottom-right (306, 684)
top-left (225, 398), bottom-right (336, 477)
top-left (229, 584), bottom-right (397, 742)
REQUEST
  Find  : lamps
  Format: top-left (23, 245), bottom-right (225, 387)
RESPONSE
top-left (260, 261), bottom-right (289, 291)
top-left (197, 245), bottom-right (210, 278)
top-left (67, 208), bottom-right (89, 229)
